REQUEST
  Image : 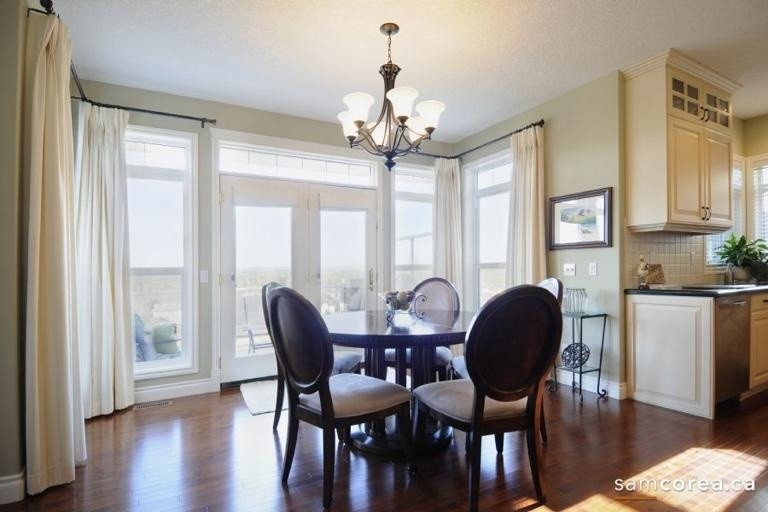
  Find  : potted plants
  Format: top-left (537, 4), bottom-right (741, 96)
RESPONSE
top-left (716, 232), bottom-right (768, 280)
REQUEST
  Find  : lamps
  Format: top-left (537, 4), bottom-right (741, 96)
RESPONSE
top-left (337, 23), bottom-right (445, 171)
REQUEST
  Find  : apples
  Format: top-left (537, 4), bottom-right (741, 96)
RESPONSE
top-left (388, 291), bottom-right (415, 310)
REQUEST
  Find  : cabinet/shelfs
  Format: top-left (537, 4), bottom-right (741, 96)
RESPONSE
top-left (624, 66), bottom-right (734, 231)
top-left (548, 314), bottom-right (608, 403)
top-left (750, 293), bottom-right (768, 397)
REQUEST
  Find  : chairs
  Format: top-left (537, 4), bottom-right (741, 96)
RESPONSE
top-left (268, 286), bottom-right (412, 509)
top-left (383, 277), bottom-right (461, 383)
top-left (449, 278), bottom-right (563, 453)
top-left (410, 284), bottom-right (562, 512)
top-left (261, 282), bottom-right (361, 440)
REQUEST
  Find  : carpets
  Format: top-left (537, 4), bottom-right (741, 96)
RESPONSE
top-left (240, 379), bottom-right (289, 415)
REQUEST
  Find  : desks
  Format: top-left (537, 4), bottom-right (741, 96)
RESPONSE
top-left (323, 310), bottom-right (467, 457)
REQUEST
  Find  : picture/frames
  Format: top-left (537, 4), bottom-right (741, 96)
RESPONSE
top-left (548, 187), bottom-right (613, 250)
